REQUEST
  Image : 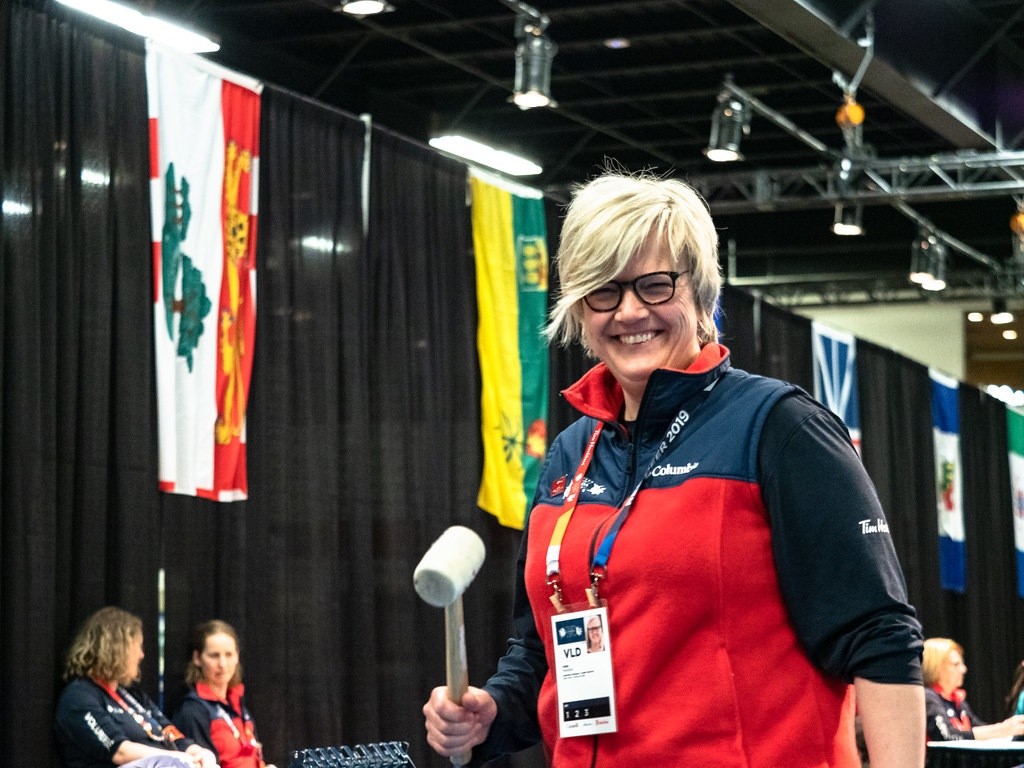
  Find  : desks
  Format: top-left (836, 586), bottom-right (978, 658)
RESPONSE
top-left (926, 737), bottom-right (1024, 768)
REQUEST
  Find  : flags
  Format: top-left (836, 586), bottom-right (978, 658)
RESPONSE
top-left (144, 39), bottom-right (266, 502)
top-left (1004, 403), bottom-right (1024, 594)
top-left (924, 364), bottom-right (968, 598)
top-left (811, 319), bottom-right (863, 463)
top-left (460, 167), bottom-right (552, 530)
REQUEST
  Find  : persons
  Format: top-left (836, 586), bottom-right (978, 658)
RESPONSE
top-left (53, 604), bottom-right (224, 768)
top-left (423, 171), bottom-right (928, 767)
top-left (921, 637), bottom-right (1023, 742)
top-left (170, 620), bottom-right (278, 768)
top-left (585, 615), bottom-right (607, 655)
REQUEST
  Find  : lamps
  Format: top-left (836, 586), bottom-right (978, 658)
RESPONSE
top-left (703, 101), bottom-right (746, 162)
top-left (333, 0), bottom-right (395, 20)
top-left (921, 243), bottom-right (948, 291)
top-left (906, 240), bottom-right (935, 285)
top-left (507, 38), bottom-right (558, 111)
top-left (829, 204), bottom-right (866, 236)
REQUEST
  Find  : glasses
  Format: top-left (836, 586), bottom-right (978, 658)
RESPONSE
top-left (583, 268), bottom-right (691, 313)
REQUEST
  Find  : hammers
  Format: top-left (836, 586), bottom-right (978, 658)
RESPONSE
top-left (413, 525), bottom-right (487, 767)
